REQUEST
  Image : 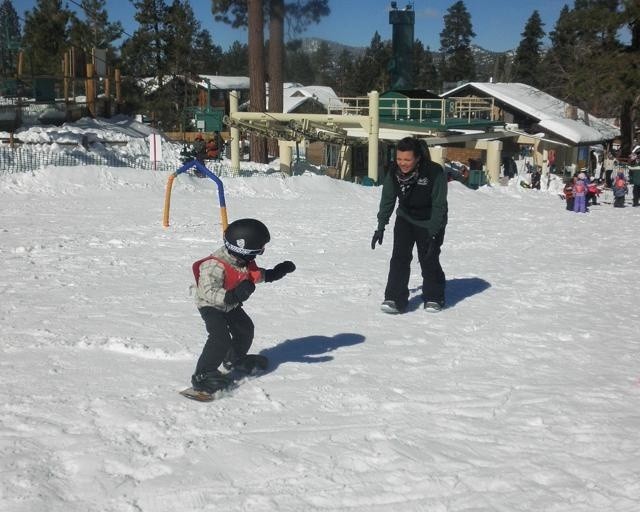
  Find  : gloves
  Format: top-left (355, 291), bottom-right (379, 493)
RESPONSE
top-left (265, 261), bottom-right (297, 283)
top-left (425, 238), bottom-right (441, 258)
top-left (371, 229), bottom-right (384, 250)
top-left (223, 279), bottom-right (256, 305)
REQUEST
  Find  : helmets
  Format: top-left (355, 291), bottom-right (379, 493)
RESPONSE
top-left (223, 218), bottom-right (272, 260)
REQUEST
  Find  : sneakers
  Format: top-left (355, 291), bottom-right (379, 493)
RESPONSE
top-left (191, 372), bottom-right (232, 391)
top-left (379, 299), bottom-right (400, 313)
top-left (425, 301), bottom-right (442, 312)
top-left (223, 354), bottom-right (268, 370)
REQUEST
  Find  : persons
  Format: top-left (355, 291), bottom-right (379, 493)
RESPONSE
top-left (502, 137), bottom-right (640, 213)
top-left (372, 137), bottom-right (448, 313)
top-left (191, 131), bottom-right (225, 178)
top-left (468, 158), bottom-right (483, 170)
top-left (193, 219), bottom-right (296, 393)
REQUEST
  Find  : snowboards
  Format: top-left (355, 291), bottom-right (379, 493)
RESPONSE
top-left (180, 355), bottom-right (267, 402)
